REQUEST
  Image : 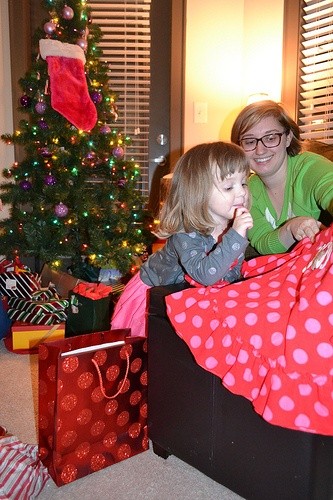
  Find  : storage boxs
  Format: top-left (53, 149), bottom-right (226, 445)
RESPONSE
top-left (11, 320), bottom-right (65, 350)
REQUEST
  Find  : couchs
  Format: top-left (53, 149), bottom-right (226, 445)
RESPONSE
top-left (146, 277), bottom-right (333, 500)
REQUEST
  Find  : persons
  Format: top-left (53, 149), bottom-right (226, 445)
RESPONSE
top-left (110, 141), bottom-right (254, 337)
top-left (231, 100), bottom-right (333, 256)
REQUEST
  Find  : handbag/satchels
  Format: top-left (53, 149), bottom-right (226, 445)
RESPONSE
top-left (36, 326), bottom-right (148, 487)
top-left (64, 282), bottom-right (113, 338)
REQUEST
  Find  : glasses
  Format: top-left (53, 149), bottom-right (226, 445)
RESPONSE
top-left (239, 131), bottom-right (286, 152)
